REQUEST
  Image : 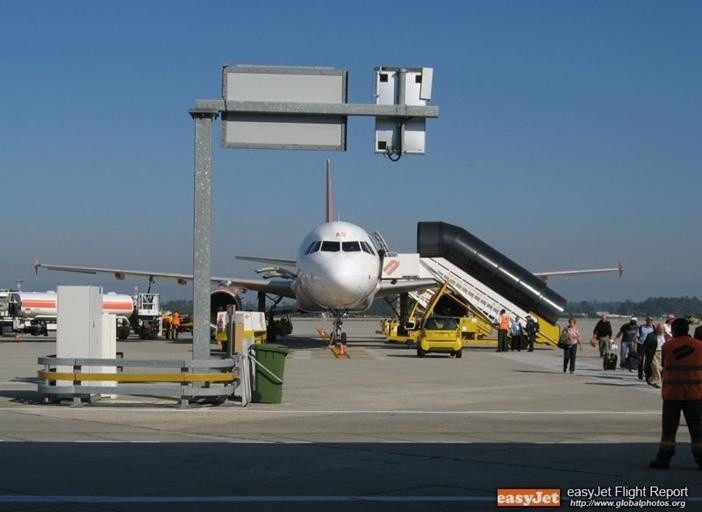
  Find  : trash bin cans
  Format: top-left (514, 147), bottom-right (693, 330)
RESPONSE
top-left (250, 344), bottom-right (288, 404)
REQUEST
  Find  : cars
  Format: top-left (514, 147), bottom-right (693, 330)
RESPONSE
top-left (413, 315), bottom-right (469, 361)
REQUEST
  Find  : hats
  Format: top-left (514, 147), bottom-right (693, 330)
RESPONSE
top-left (666, 314), bottom-right (676, 320)
top-left (524, 315), bottom-right (531, 318)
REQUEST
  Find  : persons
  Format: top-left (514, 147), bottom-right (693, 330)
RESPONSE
top-left (216, 313), bottom-right (225, 347)
top-left (510, 315), bottom-right (524, 352)
top-left (557, 318), bottom-right (583, 374)
top-left (523, 315), bottom-right (535, 352)
top-left (649, 317), bottom-right (702, 470)
top-left (164, 310), bottom-right (173, 340)
top-left (591, 314), bottom-right (701, 389)
top-left (494, 309), bottom-right (511, 352)
top-left (173, 310), bottom-right (181, 341)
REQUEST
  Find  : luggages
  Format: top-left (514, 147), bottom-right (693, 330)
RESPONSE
top-left (603, 353), bottom-right (618, 371)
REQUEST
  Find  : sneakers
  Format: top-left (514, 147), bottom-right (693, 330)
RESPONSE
top-left (649, 461), bottom-right (671, 469)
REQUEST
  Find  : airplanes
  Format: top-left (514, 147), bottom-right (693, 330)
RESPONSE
top-left (33, 154), bottom-right (632, 359)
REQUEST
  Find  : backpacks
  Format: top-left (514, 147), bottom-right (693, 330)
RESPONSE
top-left (529, 320), bottom-right (539, 332)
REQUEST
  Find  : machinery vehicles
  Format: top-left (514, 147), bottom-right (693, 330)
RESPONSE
top-left (378, 221), bottom-right (565, 348)
top-left (0, 278), bottom-right (138, 344)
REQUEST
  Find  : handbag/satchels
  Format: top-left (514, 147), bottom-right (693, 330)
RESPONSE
top-left (557, 335), bottom-right (572, 349)
top-left (608, 338), bottom-right (618, 350)
top-left (591, 337), bottom-right (598, 347)
top-left (625, 351), bottom-right (642, 369)
